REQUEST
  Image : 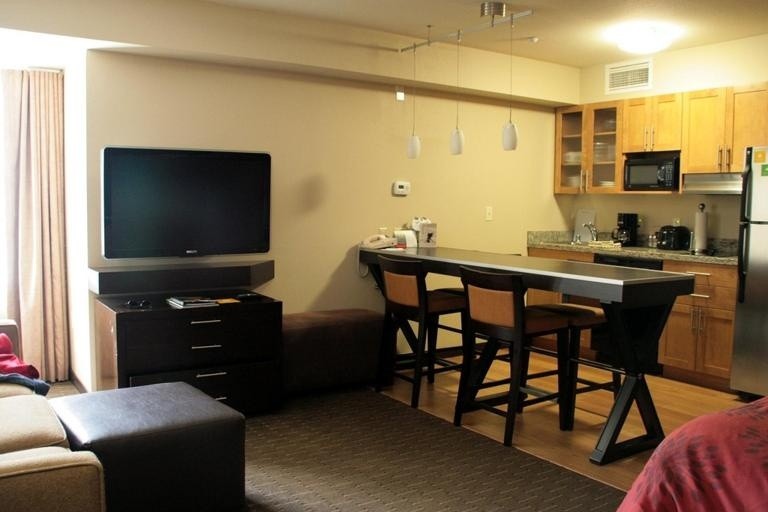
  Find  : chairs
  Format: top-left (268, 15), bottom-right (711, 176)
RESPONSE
top-left (560, 252), bottom-right (664, 433)
top-left (374, 250), bottom-right (466, 412)
top-left (452, 259), bottom-right (570, 452)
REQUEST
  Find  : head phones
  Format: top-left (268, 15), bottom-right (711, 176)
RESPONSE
top-left (125, 294), bottom-right (150, 308)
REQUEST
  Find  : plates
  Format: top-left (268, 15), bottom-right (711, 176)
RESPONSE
top-left (598, 180), bottom-right (614, 187)
top-left (594, 142), bottom-right (609, 163)
top-left (567, 177), bottom-right (584, 189)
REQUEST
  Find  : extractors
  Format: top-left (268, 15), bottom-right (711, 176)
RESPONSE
top-left (683, 172), bottom-right (743, 195)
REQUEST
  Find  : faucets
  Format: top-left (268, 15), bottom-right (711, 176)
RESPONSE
top-left (583, 222), bottom-right (597, 240)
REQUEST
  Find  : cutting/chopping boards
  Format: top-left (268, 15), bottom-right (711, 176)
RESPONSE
top-left (572, 210), bottom-right (597, 241)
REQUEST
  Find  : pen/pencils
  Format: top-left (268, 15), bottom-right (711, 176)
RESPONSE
top-left (237, 293), bottom-right (257, 297)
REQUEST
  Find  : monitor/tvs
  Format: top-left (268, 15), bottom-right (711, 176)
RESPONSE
top-left (99, 145), bottom-right (273, 267)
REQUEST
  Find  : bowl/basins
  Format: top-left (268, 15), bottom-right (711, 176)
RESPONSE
top-left (564, 151), bottom-right (581, 164)
top-left (603, 120), bottom-right (615, 131)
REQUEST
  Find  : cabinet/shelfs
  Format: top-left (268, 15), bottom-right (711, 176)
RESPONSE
top-left (523, 243), bottom-right (594, 361)
top-left (680, 80), bottom-right (766, 177)
top-left (551, 98), bottom-right (620, 197)
top-left (619, 91), bottom-right (682, 154)
top-left (655, 255), bottom-right (739, 396)
top-left (91, 289), bottom-right (287, 420)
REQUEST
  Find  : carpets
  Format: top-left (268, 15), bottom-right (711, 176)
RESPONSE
top-left (238, 392), bottom-right (630, 512)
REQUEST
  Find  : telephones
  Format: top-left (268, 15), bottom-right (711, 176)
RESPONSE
top-left (359, 235), bottom-right (398, 250)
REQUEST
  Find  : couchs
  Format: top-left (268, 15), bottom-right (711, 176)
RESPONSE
top-left (0, 318), bottom-right (108, 512)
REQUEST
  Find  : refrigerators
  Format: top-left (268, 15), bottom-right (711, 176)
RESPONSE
top-left (728, 146), bottom-right (768, 397)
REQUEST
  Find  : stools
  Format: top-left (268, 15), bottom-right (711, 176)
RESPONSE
top-left (47, 380), bottom-right (247, 511)
top-left (281, 306), bottom-right (396, 397)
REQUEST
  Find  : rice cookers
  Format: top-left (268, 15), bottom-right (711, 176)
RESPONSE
top-left (656, 225), bottom-right (690, 250)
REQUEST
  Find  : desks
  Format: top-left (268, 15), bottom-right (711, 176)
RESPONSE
top-left (356, 246), bottom-right (696, 466)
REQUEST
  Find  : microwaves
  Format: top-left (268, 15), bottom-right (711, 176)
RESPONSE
top-left (624, 159), bottom-right (678, 191)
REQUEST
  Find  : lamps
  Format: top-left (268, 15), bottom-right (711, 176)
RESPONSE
top-left (397, 9), bottom-right (531, 158)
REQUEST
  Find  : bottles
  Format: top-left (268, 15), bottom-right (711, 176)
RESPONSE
top-left (649, 235), bottom-right (657, 248)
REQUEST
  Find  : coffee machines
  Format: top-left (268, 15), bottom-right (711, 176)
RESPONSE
top-left (613, 213), bottom-right (638, 247)
